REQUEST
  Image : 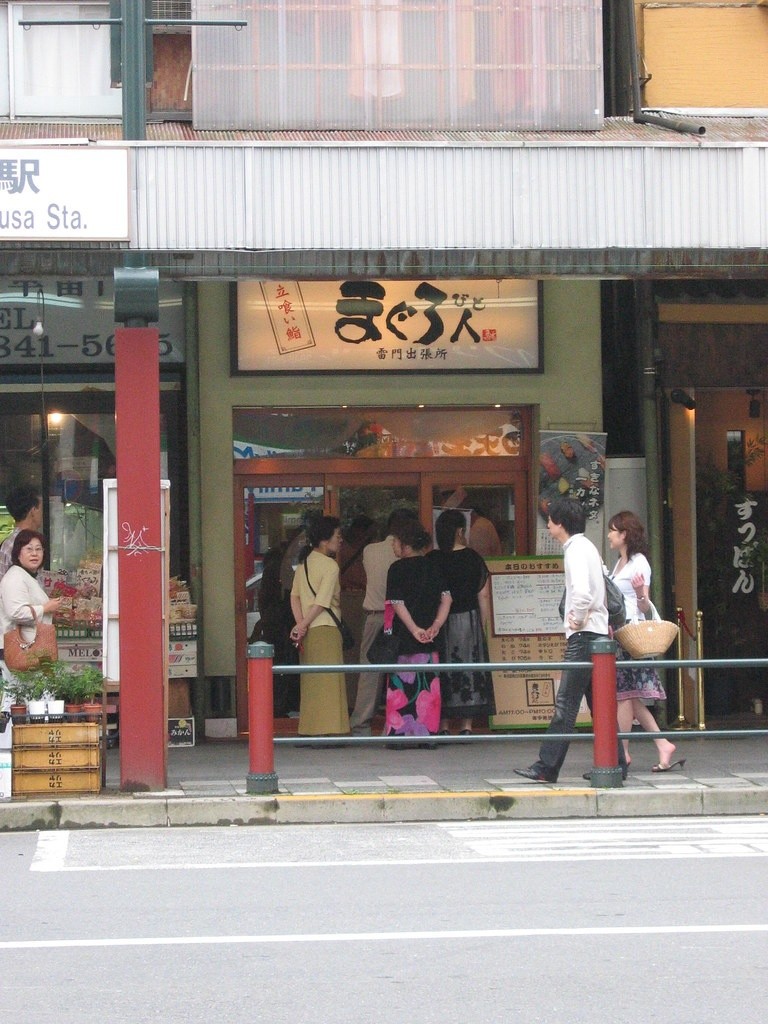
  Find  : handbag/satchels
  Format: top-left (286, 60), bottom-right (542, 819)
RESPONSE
top-left (338, 617), bottom-right (354, 651)
top-left (250, 618), bottom-right (283, 650)
top-left (612, 599), bottom-right (680, 658)
top-left (4, 604), bottom-right (58, 675)
top-left (366, 623), bottom-right (399, 664)
top-left (557, 566), bottom-right (627, 627)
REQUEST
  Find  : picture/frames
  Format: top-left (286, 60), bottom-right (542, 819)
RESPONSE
top-left (228, 279), bottom-right (547, 375)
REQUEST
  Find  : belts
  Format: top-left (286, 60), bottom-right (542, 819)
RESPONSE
top-left (367, 611), bottom-right (385, 614)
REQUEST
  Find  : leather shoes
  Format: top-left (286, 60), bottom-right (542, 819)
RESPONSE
top-left (513, 762), bottom-right (556, 782)
top-left (583, 765), bottom-right (626, 779)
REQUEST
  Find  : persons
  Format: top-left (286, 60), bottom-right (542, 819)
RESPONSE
top-left (382, 522), bottom-right (452, 751)
top-left (289, 516), bottom-right (350, 747)
top-left (0, 486), bottom-right (64, 724)
top-left (607, 511), bottom-right (686, 771)
top-left (259, 508), bottom-right (501, 634)
top-left (349, 509), bottom-right (420, 742)
top-left (513, 500), bottom-right (628, 781)
top-left (425, 510), bottom-right (491, 745)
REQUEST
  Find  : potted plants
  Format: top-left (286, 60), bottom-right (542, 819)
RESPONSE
top-left (0, 658), bottom-right (109, 724)
top-left (662, 431), bottom-right (768, 716)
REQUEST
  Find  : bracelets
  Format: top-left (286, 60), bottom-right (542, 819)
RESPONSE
top-left (637, 596), bottom-right (645, 601)
top-left (434, 619), bottom-right (443, 626)
top-left (574, 622), bottom-right (581, 626)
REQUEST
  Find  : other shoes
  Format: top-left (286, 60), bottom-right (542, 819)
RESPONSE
top-left (458, 729), bottom-right (473, 736)
top-left (437, 730), bottom-right (451, 745)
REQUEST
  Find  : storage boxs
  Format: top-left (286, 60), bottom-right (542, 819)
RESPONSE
top-left (614, 593), bottom-right (680, 661)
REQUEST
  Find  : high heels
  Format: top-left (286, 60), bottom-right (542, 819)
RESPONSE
top-left (652, 758), bottom-right (685, 771)
